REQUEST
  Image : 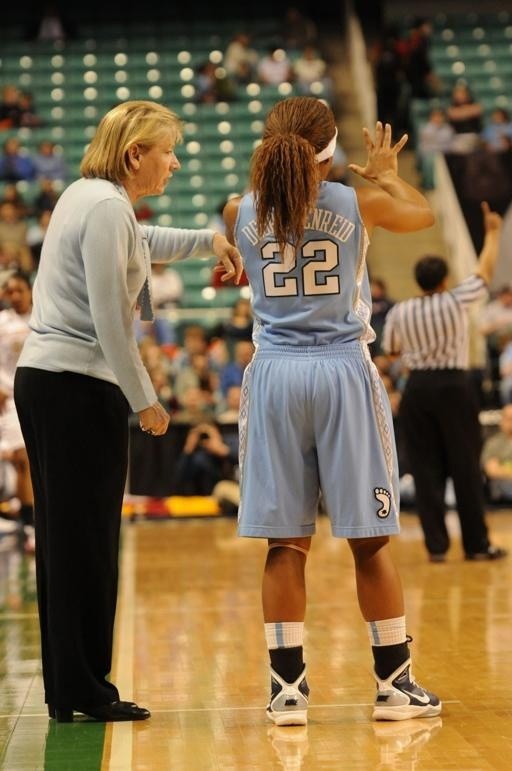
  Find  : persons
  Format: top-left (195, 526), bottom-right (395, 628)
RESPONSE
top-left (14, 100), bottom-right (242, 721)
top-left (380, 202), bottom-right (506, 561)
top-left (0, 16), bottom-right (512, 552)
top-left (224, 96), bottom-right (442, 726)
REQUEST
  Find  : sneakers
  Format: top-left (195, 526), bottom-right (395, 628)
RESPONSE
top-left (0, 515), bottom-right (36, 553)
top-left (265, 662), bottom-right (309, 726)
top-left (430, 552), bottom-right (446, 562)
top-left (465, 546), bottom-right (507, 561)
top-left (372, 647), bottom-right (442, 721)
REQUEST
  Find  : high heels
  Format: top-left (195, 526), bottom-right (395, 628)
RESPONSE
top-left (48, 700), bottom-right (151, 723)
